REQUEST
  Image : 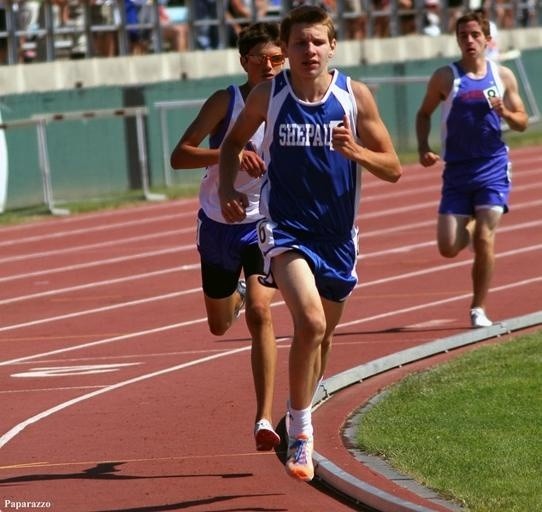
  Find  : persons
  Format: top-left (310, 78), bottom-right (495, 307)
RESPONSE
top-left (0, 0), bottom-right (541, 65)
top-left (217, 4), bottom-right (404, 483)
top-left (168, 22), bottom-right (287, 452)
top-left (413, 8), bottom-right (530, 328)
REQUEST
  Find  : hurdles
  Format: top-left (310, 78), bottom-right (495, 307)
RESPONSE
top-left (0, 52), bottom-right (537, 217)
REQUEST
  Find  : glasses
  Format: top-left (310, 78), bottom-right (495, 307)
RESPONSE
top-left (247, 55), bottom-right (285, 65)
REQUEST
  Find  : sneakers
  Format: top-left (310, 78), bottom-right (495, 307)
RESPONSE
top-left (254, 419), bottom-right (314, 481)
top-left (470, 307), bottom-right (492, 327)
